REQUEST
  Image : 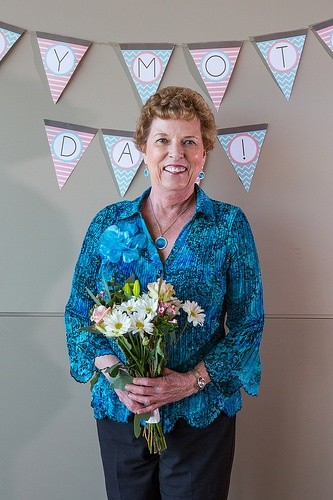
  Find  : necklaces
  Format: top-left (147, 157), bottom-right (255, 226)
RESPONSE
top-left (148, 192), bottom-right (195, 249)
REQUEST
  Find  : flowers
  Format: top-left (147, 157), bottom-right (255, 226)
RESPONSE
top-left (82, 276), bottom-right (206, 454)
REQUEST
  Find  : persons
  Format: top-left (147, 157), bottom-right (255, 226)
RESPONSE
top-left (65, 86), bottom-right (266, 500)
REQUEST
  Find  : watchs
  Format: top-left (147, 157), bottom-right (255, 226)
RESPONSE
top-left (191, 368), bottom-right (206, 390)
